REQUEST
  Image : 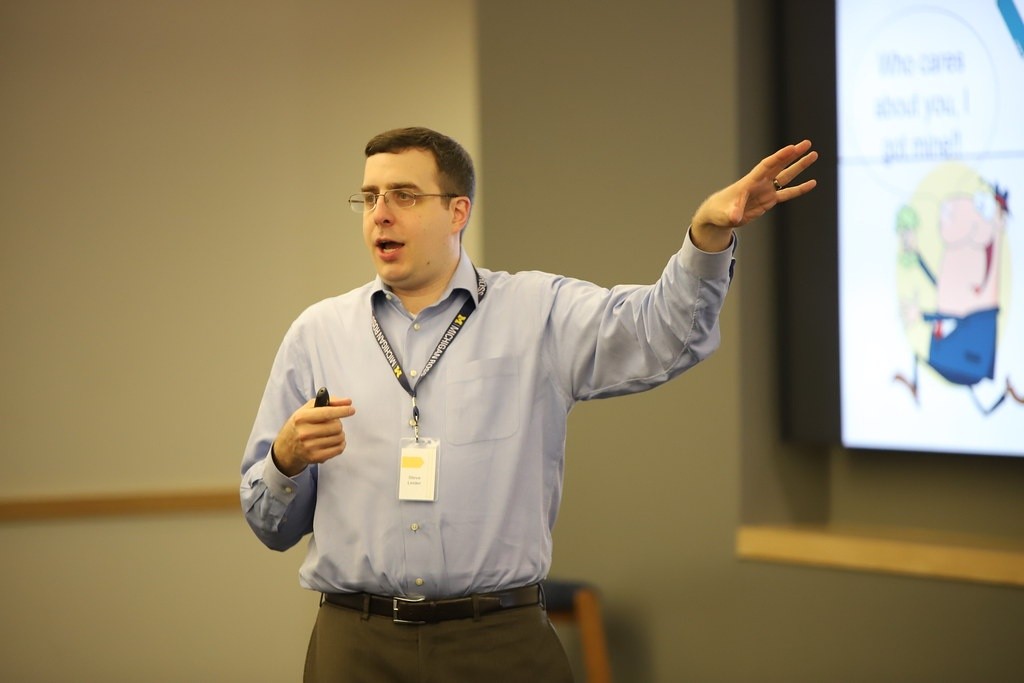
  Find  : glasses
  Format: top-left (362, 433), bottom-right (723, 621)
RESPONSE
top-left (347, 189), bottom-right (465, 214)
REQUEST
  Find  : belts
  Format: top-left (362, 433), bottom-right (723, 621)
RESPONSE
top-left (325, 584), bottom-right (542, 625)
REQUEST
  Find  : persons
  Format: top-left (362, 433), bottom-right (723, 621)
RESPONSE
top-left (240, 126), bottom-right (818, 683)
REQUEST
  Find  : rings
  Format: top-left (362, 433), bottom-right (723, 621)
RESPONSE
top-left (774, 177), bottom-right (782, 190)
